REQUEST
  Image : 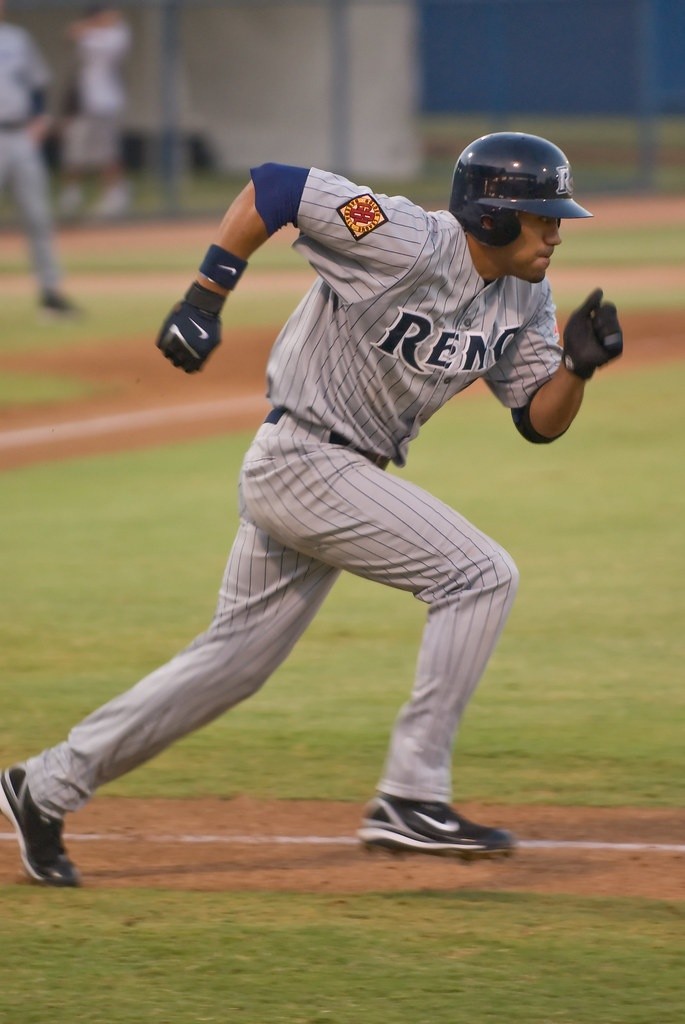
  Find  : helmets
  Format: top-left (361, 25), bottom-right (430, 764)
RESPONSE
top-left (447, 132), bottom-right (593, 244)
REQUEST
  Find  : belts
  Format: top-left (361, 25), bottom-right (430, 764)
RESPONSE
top-left (262, 409), bottom-right (389, 471)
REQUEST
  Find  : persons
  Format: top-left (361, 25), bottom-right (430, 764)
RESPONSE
top-left (0, 133), bottom-right (626, 893)
top-left (0, 0), bottom-right (130, 311)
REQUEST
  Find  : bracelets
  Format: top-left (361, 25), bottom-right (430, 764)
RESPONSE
top-left (198, 244), bottom-right (248, 292)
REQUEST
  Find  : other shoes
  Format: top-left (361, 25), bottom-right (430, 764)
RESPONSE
top-left (44, 292), bottom-right (72, 313)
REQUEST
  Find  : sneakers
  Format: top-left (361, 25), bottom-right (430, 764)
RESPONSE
top-left (356, 793), bottom-right (514, 862)
top-left (0, 767), bottom-right (78, 885)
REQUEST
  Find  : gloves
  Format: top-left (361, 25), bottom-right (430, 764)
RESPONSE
top-left (157, 283), bottom-right (226, 371)
top-left (560, 288), bottom-right (623, 381)
